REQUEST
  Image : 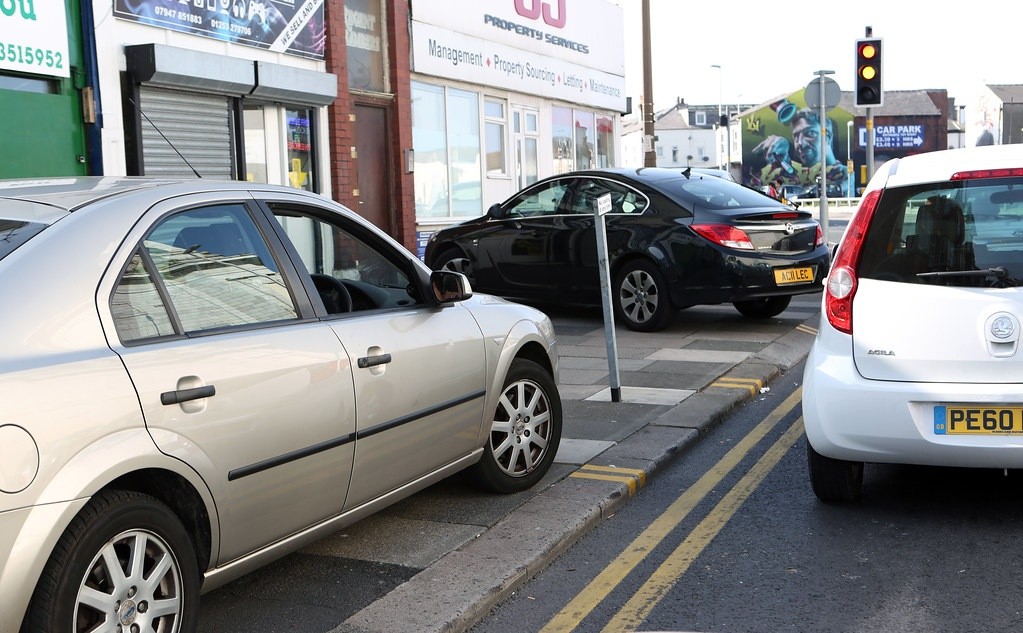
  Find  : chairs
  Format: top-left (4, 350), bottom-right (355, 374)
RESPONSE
top-left (584, 188), bottom-right (614, 213)
top-left (167, 224), bottom-right (243, 285)
top-left (907, 197), bottom-right (979, 282)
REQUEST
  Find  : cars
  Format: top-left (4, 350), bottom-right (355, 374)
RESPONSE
top-left (760, 185), bottom-right (805, 201)
top-left (801, 143), bottom-right (1023, 505)
top-left (947, 186), bottom-right (1023, 254)
top-left (424, 166), bottom-right (835, 334)
top-left (0, 176), bottom-right (565, 632)
top-left (794, 183), bottom-right (844, 201)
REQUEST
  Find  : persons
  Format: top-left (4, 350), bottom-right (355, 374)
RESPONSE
top-left (751, 108), bottom-right (855, 198)
top-left (768, 179), bottom-right (787, 201)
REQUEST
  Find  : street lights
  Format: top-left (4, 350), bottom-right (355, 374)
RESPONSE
top-left (813, 68), bottom-right (838, 231)
top-left (846, 121), bottom-right (856, 205)
top-left (708, 64), bottom-right (724, 170)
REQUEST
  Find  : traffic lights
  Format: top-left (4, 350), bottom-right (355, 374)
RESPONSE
top-left (854, 35), bottom-right (886, 109)
